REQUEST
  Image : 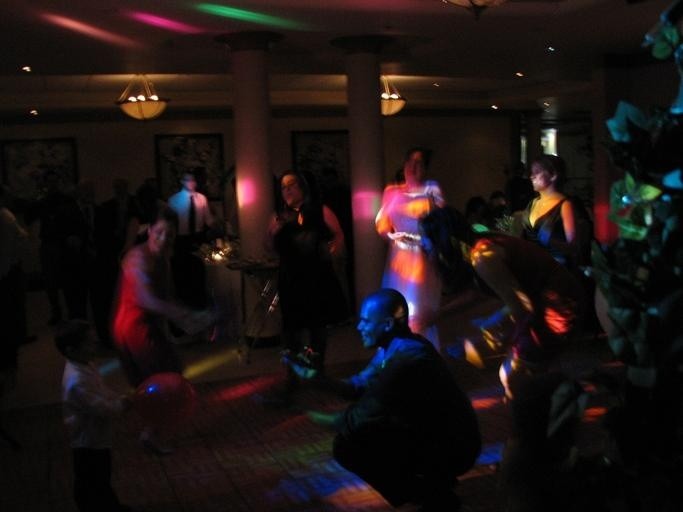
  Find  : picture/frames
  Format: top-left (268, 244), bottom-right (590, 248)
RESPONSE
top-left (0, 137), bottom-right (78, 213)
top-left (153, 132), bottom-right (225, 203)
top-left (289, 129), bottom-right (349, 211)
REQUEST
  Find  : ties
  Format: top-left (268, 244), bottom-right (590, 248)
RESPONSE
top-left (188, 196), bottom-right (197, 235)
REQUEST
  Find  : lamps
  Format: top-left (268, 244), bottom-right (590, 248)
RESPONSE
top-left (379, 75), bottom-right (406, 116)
top-left (115, 73), bottom-right (170, 120)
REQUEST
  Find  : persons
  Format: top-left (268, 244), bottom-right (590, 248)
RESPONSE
top-left (3, 162), bottom-right (167, 396)
top-left (593, 108), bottom-right (683, 511)
top-left (407, 203), bottom-right (590, 483)
top-left (374, 147), bottom-right (447, 339)
top-left (58, 330), bottom-right (142, 512)
top-left (109, 205), bottom-right (217, 456)
top-left (469, 156), bottom-right (591, 270)
top-left (261, 170), bottom-right (344, 373)
top-left (167, 170), bottom-right (214, 235)
top-left (286, 286), bottom-right (484, 512)
top-left (52, 322), bottom-right (142, 512)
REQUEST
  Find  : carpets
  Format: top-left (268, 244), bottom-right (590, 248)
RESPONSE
top-left (2, 353), bottom-right (543, 510)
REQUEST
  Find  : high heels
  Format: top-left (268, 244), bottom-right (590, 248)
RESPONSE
top-left (139, 431), bottom-right (173, 455)
